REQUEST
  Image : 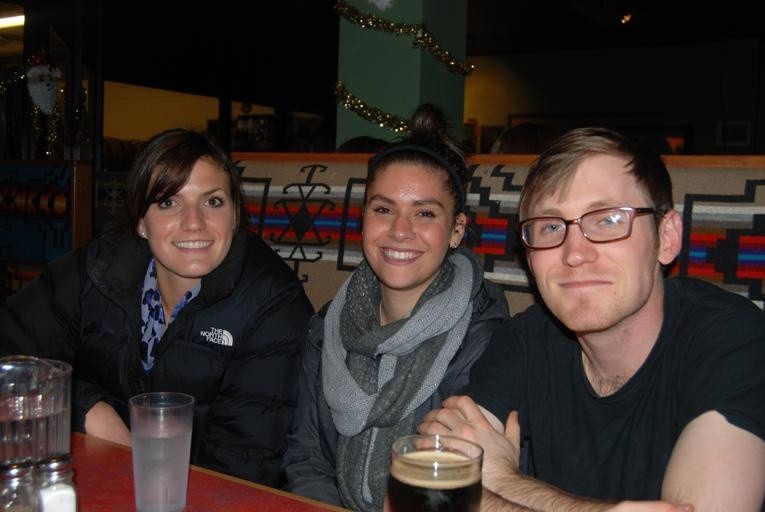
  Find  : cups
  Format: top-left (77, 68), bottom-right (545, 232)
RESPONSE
top-left (387, 432), bottom-right (484, 510)
top-left (0, 356), bottom-right (73, 478)
top-left (129, 391), bottom-right (195, 511)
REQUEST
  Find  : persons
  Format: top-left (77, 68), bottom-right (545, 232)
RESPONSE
top-left (406, 126), bottom-right (765, 510)
top-left (282, 139), bottom-right (512, 510)
top-left (409, 102), bottom-right (448, 147)
top-left (1, 128), bottom-right (316, 492)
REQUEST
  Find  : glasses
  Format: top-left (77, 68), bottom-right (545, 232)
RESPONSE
top-left (515, 207), bottom-right (658, 249)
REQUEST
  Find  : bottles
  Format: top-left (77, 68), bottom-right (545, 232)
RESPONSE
top-left (34, 452), bottom-right (80, 512)
top-left (0, 456), bottom-right (42, 512)
